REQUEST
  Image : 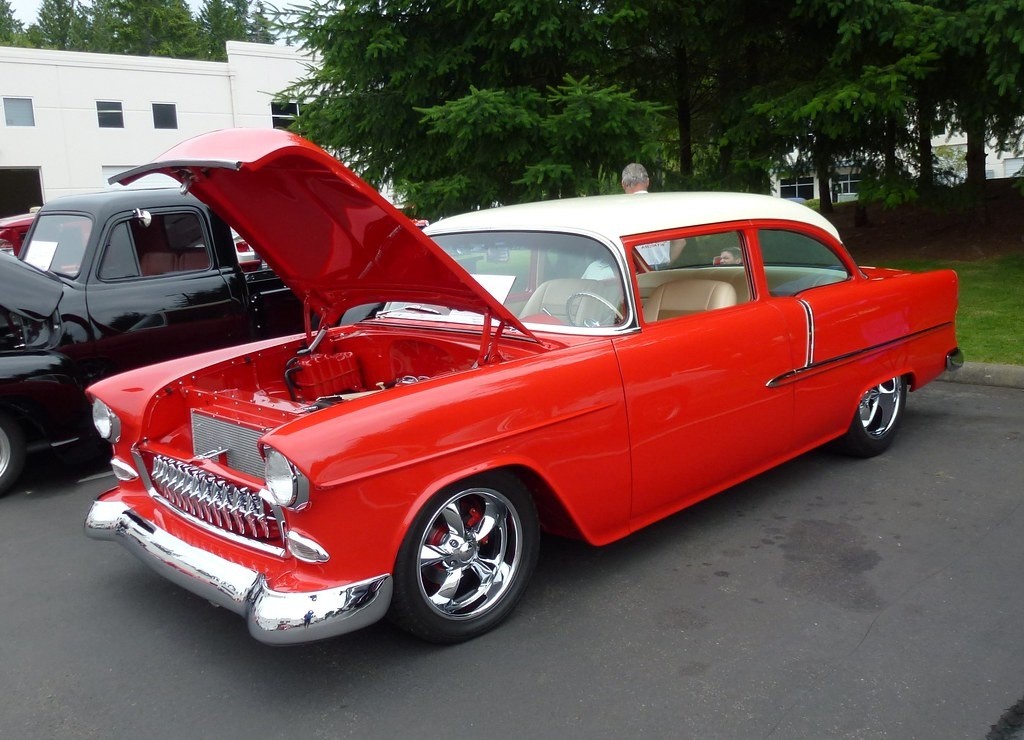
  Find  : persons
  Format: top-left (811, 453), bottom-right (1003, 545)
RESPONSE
top-left (622, 163), bottom-right (686, 270)
top-left (720, 248), bottom-right (742, 264)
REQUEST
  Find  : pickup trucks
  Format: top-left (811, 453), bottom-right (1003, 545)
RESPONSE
top-left (0, 186), bottom-right (483, 496)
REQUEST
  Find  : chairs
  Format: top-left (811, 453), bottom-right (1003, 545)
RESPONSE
top-left (642, 275), bottom-right (738, 333)
top-left (520, 270), bottom-right (611, 336)
top-left (136, 250), bottom-right (178, 276)
top-left (179, 247), bottom-right (213, 271)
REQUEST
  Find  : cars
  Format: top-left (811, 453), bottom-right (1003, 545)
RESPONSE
top-left (0, 208), bottom-right (259, 270)
top-left (81, 124), bottom-right (964, 648)
